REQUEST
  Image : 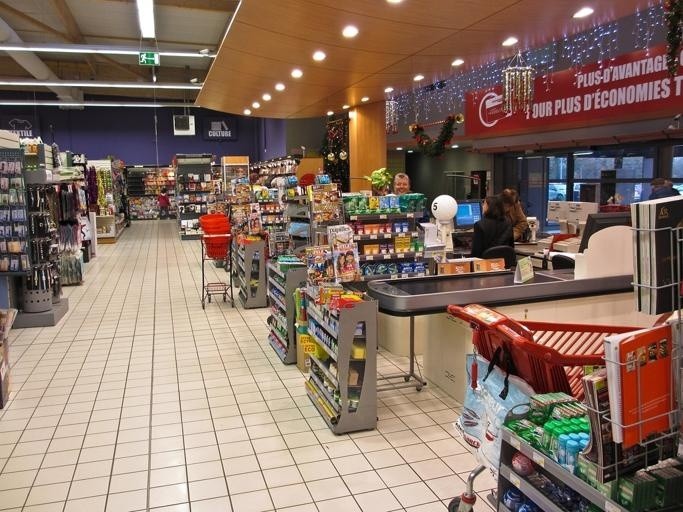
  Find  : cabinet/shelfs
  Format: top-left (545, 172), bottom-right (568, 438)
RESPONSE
top-left (236, 238), bottom-right (267, 309)
top-left (495, 404), bottom-right (682, 512)
top-left (346, 213), bottom-right (424, 281)
top-left (257, 198), bottom-right (283, 233)
top-left (302, 286), bottom-right (379, 435)
top-left (175, 179), bottom-right (213, 239)
top-left (0, 127), bottom-right (174, 329)
top-left (266, 261), bottom-right (309, 365)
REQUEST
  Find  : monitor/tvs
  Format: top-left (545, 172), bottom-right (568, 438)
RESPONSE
top-left (454, 199), bottom-right (482, 229)
top-left (578, 212), bottom-right (630, 251)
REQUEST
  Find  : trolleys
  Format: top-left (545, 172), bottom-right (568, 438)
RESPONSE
top-left (442, 304), bottom-right (654, 512)
top-left (200, 212), bottom-right (235, 309)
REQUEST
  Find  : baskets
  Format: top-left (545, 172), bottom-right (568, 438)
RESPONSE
top-left (199, 214), bottom-right (233, 258)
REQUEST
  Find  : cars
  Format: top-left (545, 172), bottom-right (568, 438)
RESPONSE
top-left (574, 183), bottom-right (581, 201)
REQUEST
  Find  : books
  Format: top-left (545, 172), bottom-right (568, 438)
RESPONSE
top-left (305, 183), bottom-right (362, 291)
top-left (602, 325), bottom-right (675, 447)
top-left (580, 367), bottom-right (665, 483)
top-left (628, 195), bottom-right (683, 315)
top-left (245, 202), bottom-right (266, 236)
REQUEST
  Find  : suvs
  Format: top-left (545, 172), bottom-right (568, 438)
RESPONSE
top-left (548, 182), bottom-right (566, 201)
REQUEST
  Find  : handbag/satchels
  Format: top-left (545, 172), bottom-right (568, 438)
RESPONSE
top-left (454, 341), bottom-right (539, 449)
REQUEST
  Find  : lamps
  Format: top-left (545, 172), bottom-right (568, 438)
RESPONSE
top-left (136, 0), bottom-right (155, 39)
top-left (187, 42), bottom-right (210, 87)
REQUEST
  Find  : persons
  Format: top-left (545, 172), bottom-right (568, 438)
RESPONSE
top-left (157, 188), bottom-right (171, 219)
top-left (648, 177), bottom-right (679, 199)
top-left (497, 188), bottom-right (527, 241)
top-left (383, 171), bottom-right (410, 196)
top-left (322, 251), bottom-right (359, 283)
top-left (116, 160), bottom-right (131, 227)
top-left (663, 179), bottom-right (680, 196)
top-left (471, 196), bottom-right (518, 268)
top-left (247, 203), bottom-right (260, 234)
